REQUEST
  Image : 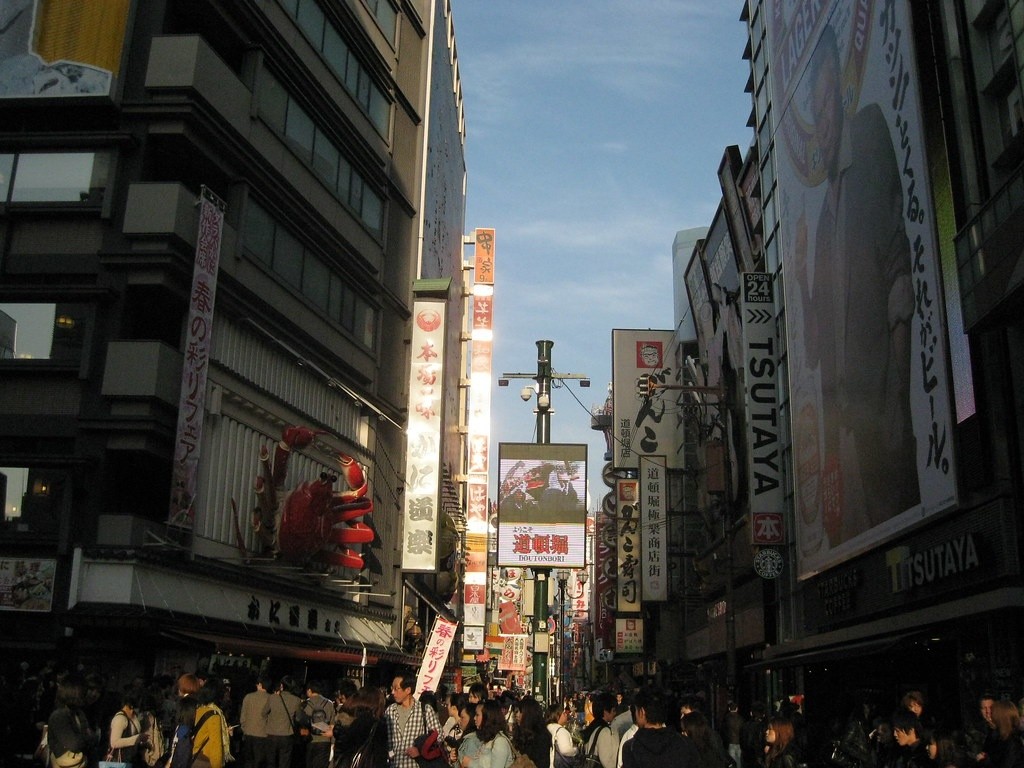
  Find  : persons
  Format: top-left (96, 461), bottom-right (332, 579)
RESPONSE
top-left (902, 692), bottom-right (936, 730)
top-left (543, 705), bottom-right (578, 768)
top-left (876, 722), bottom-right (900, 768)
top-left (739, 705), bottom-right (767, 768)
top-left (189, 679), bottom-right (235, 768)
top-left (763, 717), bottom-right (807, 768)
top-left (790, 25), bottom-right (917, 550)
top-left (510, 697), bottom-right (552, 768)
top-left (0, 659), bottom-right (180, 768)
top-left (462, 700), bottom-right (514, 768)
top-left (679, 695), bottom-right (723, 745)
top-left (892, 710), bottom-right (932, 768)
top-left (333, 687), bottom-right (390, 768)
top-left (176, 698), bottom-right (196, 739)
top-left (926, 728), bottom-right (960, 768)
top-left (622, 694), bottom-right (703, 768)
top-left (261, 676), bottom-right (305, 768)
top-left (681, 711), bottom-right (727, 767)
top-left (975, 699), bottom-right (1024, 768)
top-left (841, 699), bottom-right (879, 767)
top-left (384, 674), bottom-right (443, 768)
top-left (978, 694), bottom-right (998, 754)
top-left (722, 704), bottom-right (743, 767)
top-left (177, 674), bottom-right (199, 700)
top-left (240, 676), bottom-right (272, 768)
top-left (445, 703), bottom-right (476, 754)
top-left (412, 680), bottom-right (627, 736)
top-left (579, 693), bottom-right (624, 768)
top-left (300, 683), bottom-right (335, 767)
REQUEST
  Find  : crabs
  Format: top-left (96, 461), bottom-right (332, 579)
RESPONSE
top-left (253, 423), bottom-right (375, 569)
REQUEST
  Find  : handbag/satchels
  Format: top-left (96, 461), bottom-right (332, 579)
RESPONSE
top-left (491, 735), bottom-right (537, 768)
top-left (553, 727), bottom-right (585, 768)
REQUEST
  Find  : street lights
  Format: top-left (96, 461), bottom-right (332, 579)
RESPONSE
top-left (635, 373), bottom-right (741, 709)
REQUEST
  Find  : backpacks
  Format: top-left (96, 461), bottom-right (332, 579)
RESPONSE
top-left (573, 726), bottom-right (604, 768)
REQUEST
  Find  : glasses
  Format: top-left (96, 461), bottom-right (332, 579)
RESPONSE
top-left (390, 685), bottom-right (405, 691)
